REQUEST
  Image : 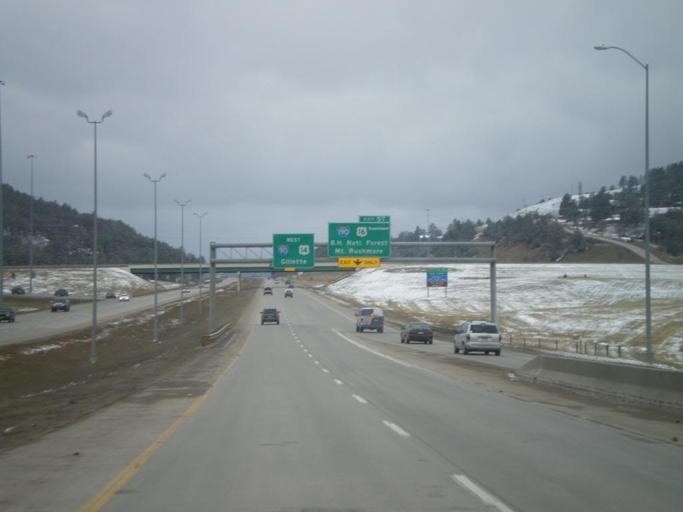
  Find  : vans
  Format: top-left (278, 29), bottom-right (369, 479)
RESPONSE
top-left (355, 308), bottom-right (383, 332)
top-left (283, 288), bottom-right (293, 297)
top-left (264, 285), bottom-right (272, 295)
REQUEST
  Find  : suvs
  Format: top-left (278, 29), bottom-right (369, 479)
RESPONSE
top-left (454, 320), bottom-right (502, 355)
top-left (260, 308), bottom-right (279, 324)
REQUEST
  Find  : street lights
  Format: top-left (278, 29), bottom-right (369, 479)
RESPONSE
top-left (143, 172), bottom-right (165, 340)
top-left (26, 153), bottom-right (37, 291)
top-left (176, 198), bottom-right (190, 326)
top-left (195, 211), bottom-right (207, 314)
top-left (76, 109), bottom-right (113, 358)
top-left (596, 42), bottom-right (651, 360)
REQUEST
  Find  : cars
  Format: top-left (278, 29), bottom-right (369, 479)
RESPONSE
top-left (400, 321), bottom-right (434, 344)
top-left (182, 287), bottom-right (189, 293)
top-left (0, 308), bottom-right (16, 322)
top-left (118, 290), bottom-right (129, 301)
top-left (51, 299), bottom-right (70, 312)
top-left (55, 289), bottom-right (68, 297)
top-left (11, 286), bottom-right (24, 294)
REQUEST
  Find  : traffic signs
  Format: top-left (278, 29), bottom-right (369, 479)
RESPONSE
top-left (272, 234), bottom-right (315, 271)
top-left (337, 258), bottom-right (379, 269)
top-left (328, 217), bottom-right (391, 259)
